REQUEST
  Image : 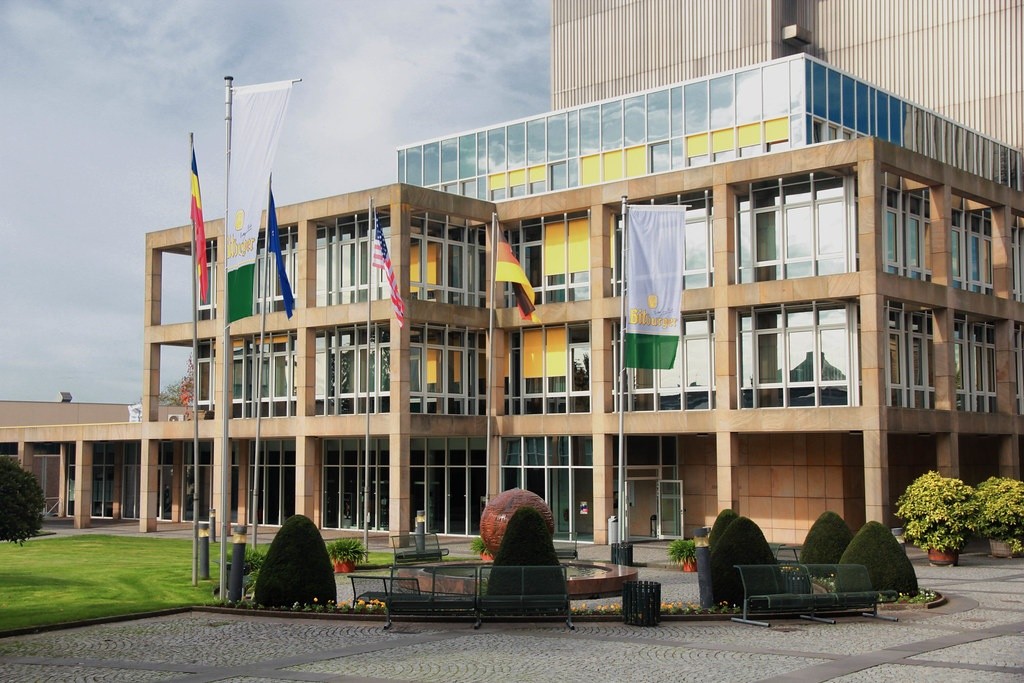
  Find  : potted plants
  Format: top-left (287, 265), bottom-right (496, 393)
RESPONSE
top-left (326, 538), bottom-right (367, 573)
top-left (471, 537), bottom-right (494, 561)
top-left (893, 470), bottom-right (986, 566)
top-left (666, 538), bottom-right (697, 572)
top-left (970, 476), bottom-right (1024, 559)
top-left (211, 545), bottom-right (264, 599)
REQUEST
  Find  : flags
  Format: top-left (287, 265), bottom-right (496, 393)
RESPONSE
top-left (372, 211), bottom-right (405, 329)
top-left (269, 192), bottom-right (295, 318)
top-left (189, 149), bottom-right (209, 305)
top-left (494, 223), bottom-right (540, 324)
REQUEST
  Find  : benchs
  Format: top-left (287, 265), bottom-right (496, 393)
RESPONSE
top-left (731, 564), bottom-right (836, 627)
top-left (384, 565), bottom-right (481, 629)
top-left (475, 566), bottom-right (574, 629)
top-left (553, 532), bottom-right (578, 560)
top-left (347, 575), bottom-right (432, 612)
top-left (769, 546), bottom-right (803, 565)
top-left (390, 534), bottom-right (449, 568)
top-left (800, 564), bottom-right (898, 624)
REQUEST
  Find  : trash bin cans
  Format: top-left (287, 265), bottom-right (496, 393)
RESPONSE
top-left (622, 580), bottom-right (662, 626)
top-left (612, 543), bottom-right (633, 566)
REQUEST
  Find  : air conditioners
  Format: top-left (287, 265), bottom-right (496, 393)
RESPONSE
top-left (168, 414), bottom-right (185, 422)
